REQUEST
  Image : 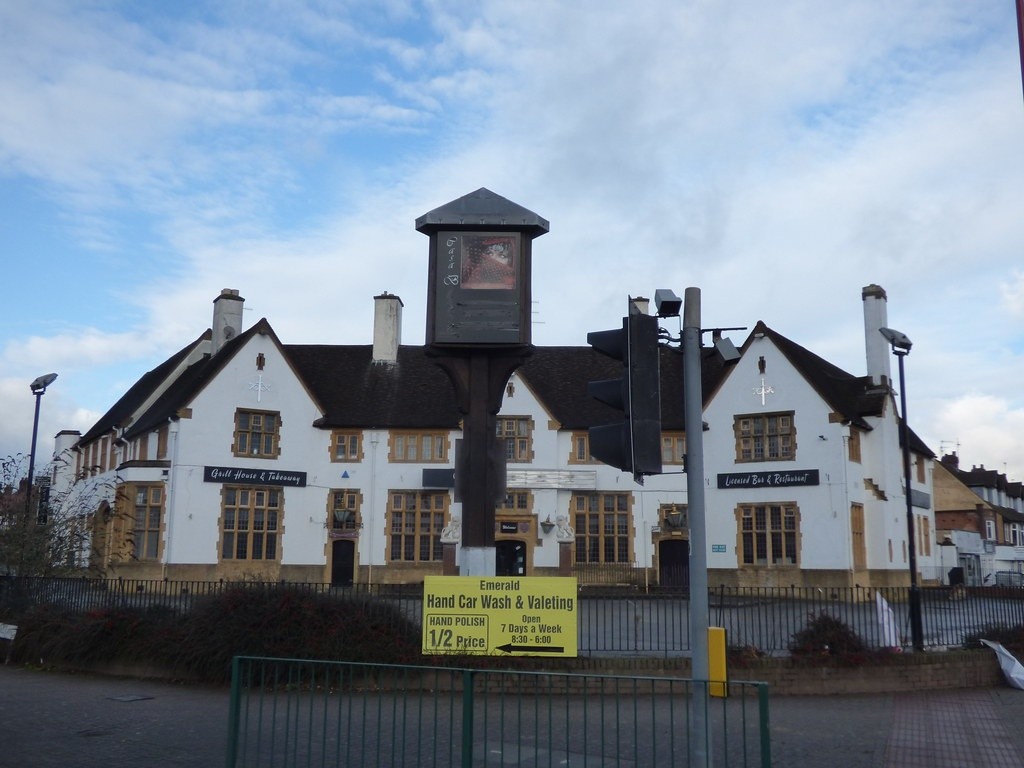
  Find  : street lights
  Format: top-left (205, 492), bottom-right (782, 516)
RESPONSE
top-left (17, 372), bottom-right (58, 580)
top-left (880, 327), bottom-right (925, 653)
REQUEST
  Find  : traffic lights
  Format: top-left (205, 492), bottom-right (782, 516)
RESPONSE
top-left (586, 294), bottom-right (663, 482)
